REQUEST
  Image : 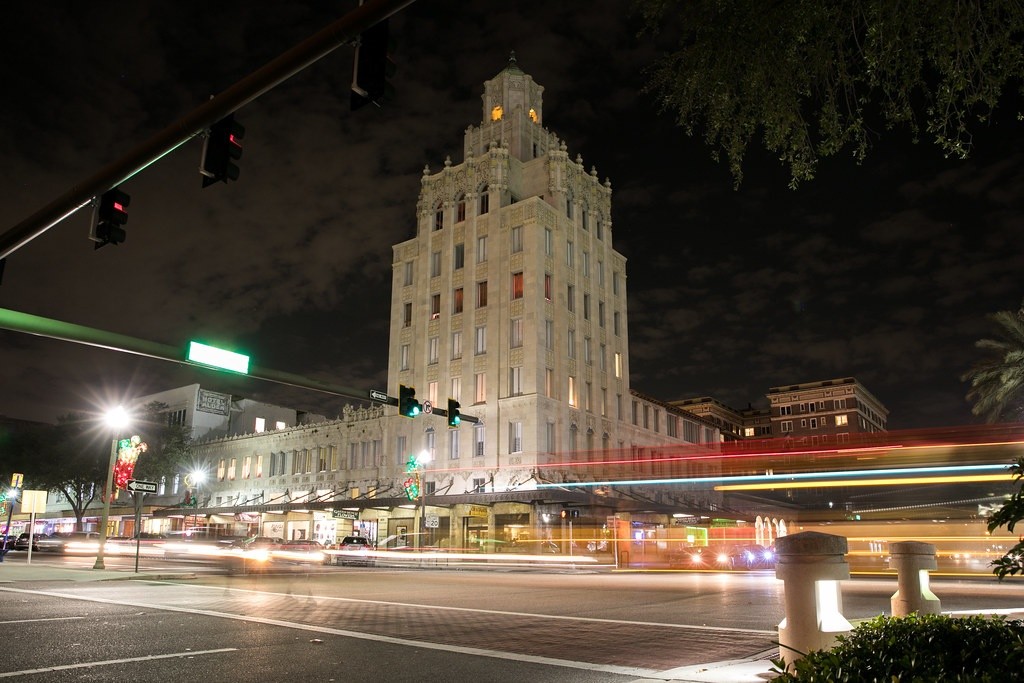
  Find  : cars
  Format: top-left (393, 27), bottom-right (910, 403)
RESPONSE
top-left (14, 532), bottom-right (53, 552)
top-left (35, 531), bottom-right (286, 557)
top-left (280, 540), bottom-right (331, 565)
top-left (337, 535), bottom-right (376, 567)
top-left (669, 547), bottom-right (730, 570)
top-left (729, 544), bottom-right (775, 570)
top-left (0, 534), bottom-right (17, 550)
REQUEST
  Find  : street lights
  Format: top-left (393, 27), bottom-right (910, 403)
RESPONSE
top-left (92, 405), bottom-right (127, 569)
top-left (193, 472), bottom-right (202, 540)
top-left (419, 450), bottom-right (430, 553)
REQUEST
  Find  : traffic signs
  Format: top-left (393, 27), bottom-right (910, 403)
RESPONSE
top-left (128, 481), bottom-right (157, 496)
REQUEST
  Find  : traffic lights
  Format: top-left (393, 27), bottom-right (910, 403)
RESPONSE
top-left (448, 397), bottom-right (461, 429)
top-left (97, 188), bottom-right (129, 249)
top-left (199, 120), bottom-right (242, 188)
top-left (398, 384), bottom-right (419, 419)
top-left (560, 510), bottom-right (570, 517)
